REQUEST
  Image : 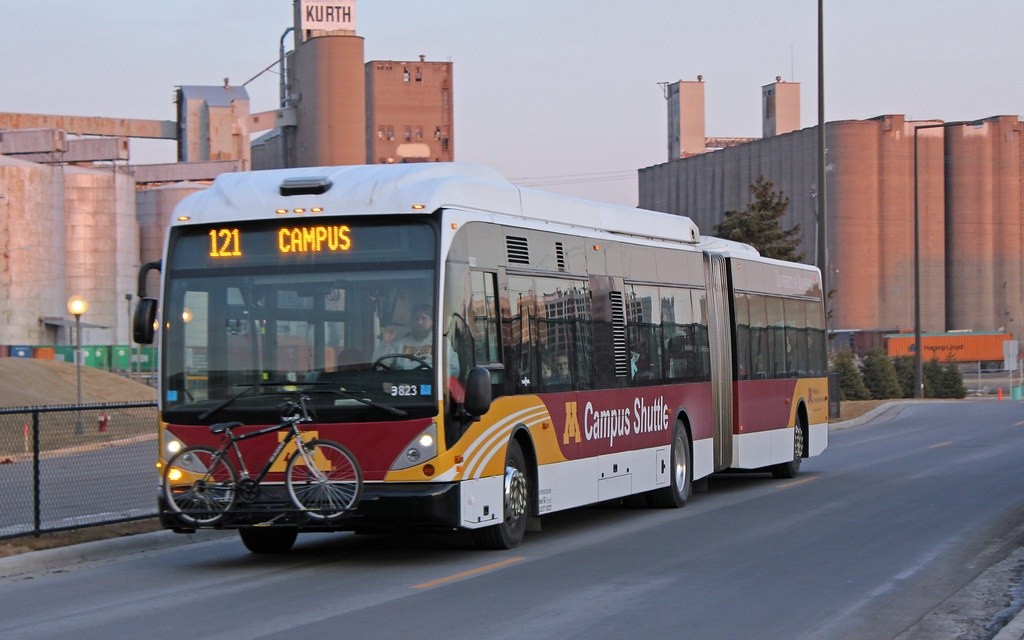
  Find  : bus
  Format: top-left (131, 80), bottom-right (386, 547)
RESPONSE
top-left (131, 161), bottom-right (830, 555)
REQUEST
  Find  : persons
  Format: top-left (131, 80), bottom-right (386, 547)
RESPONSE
top-left (374, 304), bottom-right (460, 381)
top-left (541, 352), bottom-right (575, 393)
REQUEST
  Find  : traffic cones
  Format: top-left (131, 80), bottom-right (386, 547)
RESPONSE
top-left (998, 388), bottom-right (1002, 400)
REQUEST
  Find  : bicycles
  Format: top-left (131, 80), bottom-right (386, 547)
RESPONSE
top-left (162, 397), bottom-right (364, 528)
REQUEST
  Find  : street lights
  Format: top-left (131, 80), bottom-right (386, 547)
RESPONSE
top-left (68, 294), bottom-right (87, 437)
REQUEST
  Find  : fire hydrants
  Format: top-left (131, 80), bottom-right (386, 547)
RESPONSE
top-left (98, 410), bottom-right (110, 432)
